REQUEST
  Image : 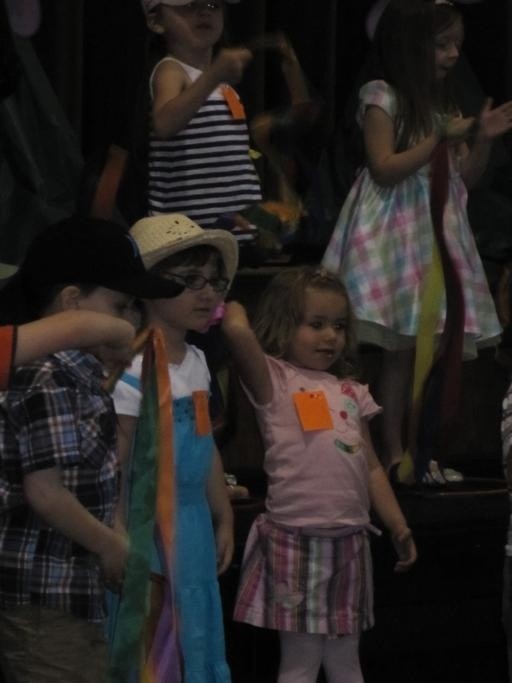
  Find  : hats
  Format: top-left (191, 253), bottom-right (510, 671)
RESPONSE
top-left (131, 214), bottom-right (239, 283)
top-left (26, 217), bottom-right (185, 298)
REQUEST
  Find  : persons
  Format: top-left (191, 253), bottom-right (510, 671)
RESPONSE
top-left (0, 0), bottom-right (512, 682)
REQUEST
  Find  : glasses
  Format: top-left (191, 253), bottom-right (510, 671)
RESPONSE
top-left (159, 267), bottom-right (231, 294)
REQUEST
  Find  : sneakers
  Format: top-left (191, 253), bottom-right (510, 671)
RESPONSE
top-left (442, 467), bottom-right (462, 483)
